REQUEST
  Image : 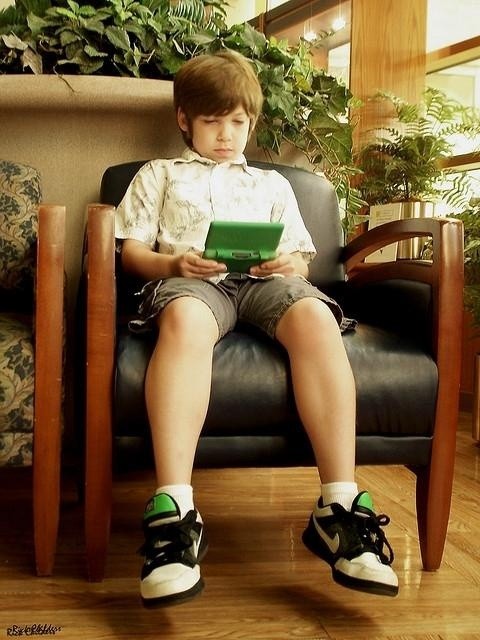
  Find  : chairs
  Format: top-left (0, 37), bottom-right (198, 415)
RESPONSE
top-left (1, 157), bottom-right (68, 577)
top-left (81, 160), bottom-right (466, 584)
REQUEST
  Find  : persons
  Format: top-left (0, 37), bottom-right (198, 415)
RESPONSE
top-left (115, 48), bottom-right (402, 608)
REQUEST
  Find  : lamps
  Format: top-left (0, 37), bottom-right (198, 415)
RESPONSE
top-left (327, 1), bottom-right (346, 33)
top-left (298, 0), bottom-right (317, 44)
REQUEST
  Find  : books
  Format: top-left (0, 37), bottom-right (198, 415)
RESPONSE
top-left (369, 199), bottom-right (438, 265)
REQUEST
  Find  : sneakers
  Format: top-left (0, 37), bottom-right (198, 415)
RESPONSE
top-left (302, 491), bottom-right (399, 597)
top-left (136, 493), bottom-right (209, 605)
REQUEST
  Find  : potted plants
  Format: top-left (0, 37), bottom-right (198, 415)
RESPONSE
top-left (422, 207), bottom-right (479, 396)
